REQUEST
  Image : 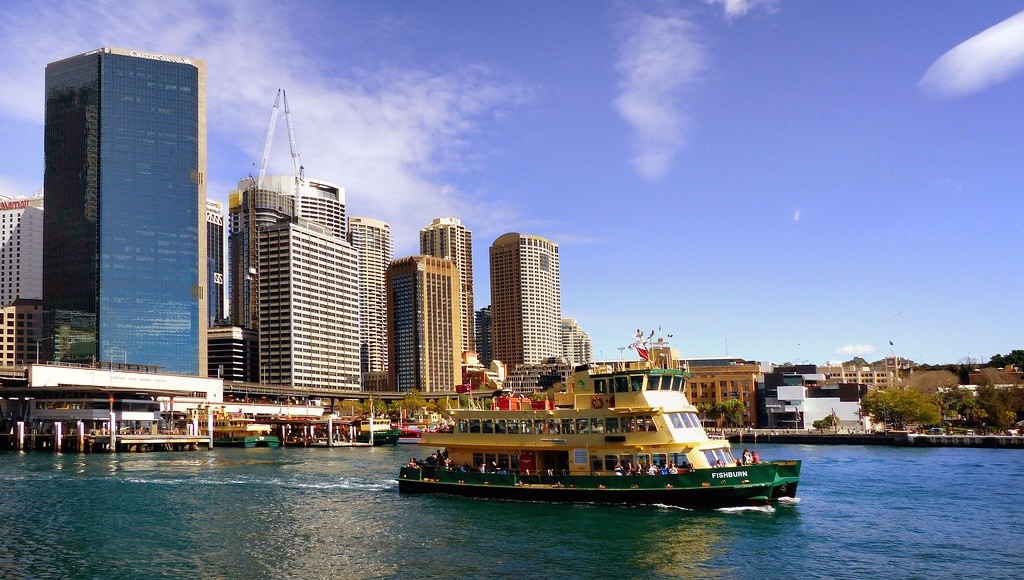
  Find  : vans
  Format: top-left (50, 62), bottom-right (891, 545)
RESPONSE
top-left (927, 428), bottom-right (943, 434)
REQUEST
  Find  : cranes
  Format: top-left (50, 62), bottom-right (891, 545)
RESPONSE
top-left (283, 91), bottom-right (305, 195)
top-left (248, 89), bottom-right (283, 190)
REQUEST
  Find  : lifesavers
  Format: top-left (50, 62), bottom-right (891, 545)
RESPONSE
top-left (591, 396), bottom-right (604, 410)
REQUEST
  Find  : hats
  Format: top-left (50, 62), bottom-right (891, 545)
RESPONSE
top-left (743, 448), bottom-right (748, 452)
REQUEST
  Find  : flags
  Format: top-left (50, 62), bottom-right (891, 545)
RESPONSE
top-left (455, 384), bottom-right (471, 393)
top-left (636, 347), bottom-right (648, 360)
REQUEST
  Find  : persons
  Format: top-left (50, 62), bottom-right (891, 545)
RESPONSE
top-left (639, 422), bottom-right (657, 431)
top-left (614, 462), bottom-right (678, 476)
top-left (716, 460), bottom-right (722, 468)
top-left (463, 461), bottom-right (469, 472)
top-left (736, 449), bottom-right (759, 466)
top-left (452, 418), bottom-right (466, 425)
top-left (439, 423), bottom-right (445, 433)
top-left (479, 461), bottom-right (501, 473)
top-left (431, 450), bottom-right (452, 471)
top-left (409, 458), bottom-right (419, 469)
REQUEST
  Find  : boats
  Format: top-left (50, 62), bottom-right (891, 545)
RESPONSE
top-left (395, 329), bottom-right (802, 505)
top-left (199, 407), bottom-right (279, 447)
top-left (344, 416), bottom-right (401, 446)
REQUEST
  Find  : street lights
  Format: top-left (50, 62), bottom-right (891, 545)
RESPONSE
top-left (397, 373), bottom-right (407, 392)
top-left (264, 362), bottom-right (275, 385)
top-left (37, 334), bottom-right (59, 363)
